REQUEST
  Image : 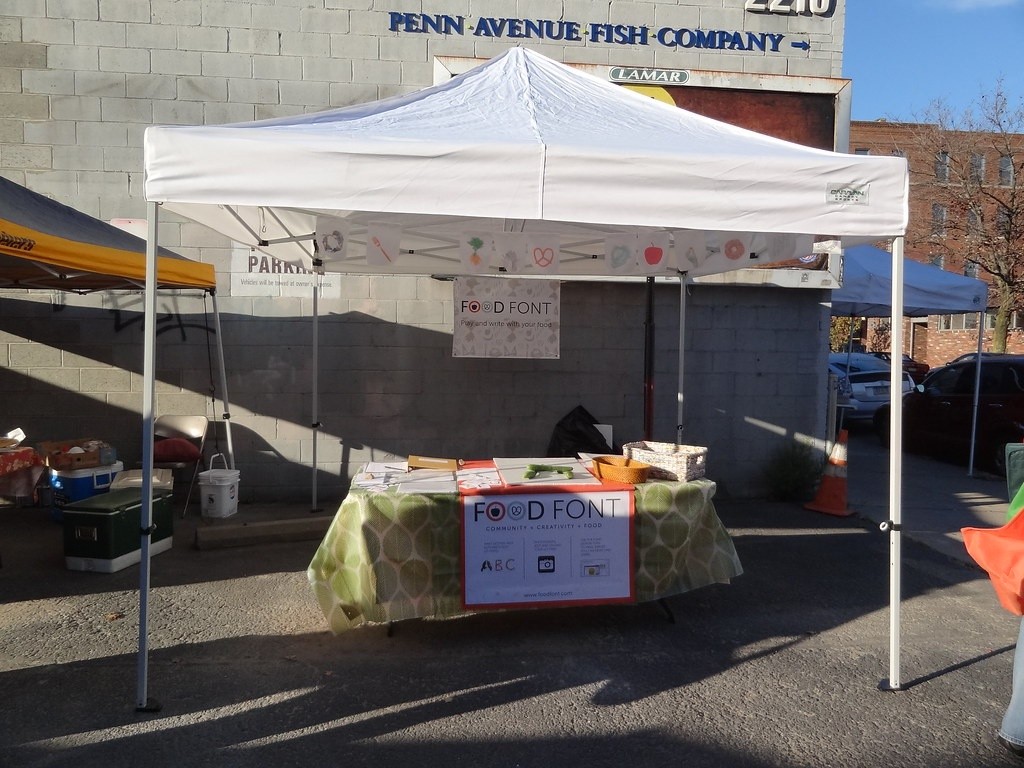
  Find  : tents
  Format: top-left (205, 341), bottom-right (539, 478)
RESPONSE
top-left (828, 243), bottom-right (988, 476)
top-left (1, 178), bottom-right (239, 521)
top-left (134, 43), bottom-right (913, 705)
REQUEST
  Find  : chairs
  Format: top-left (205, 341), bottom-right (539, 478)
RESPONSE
top-left (137, 414), bottom-right (209, 518)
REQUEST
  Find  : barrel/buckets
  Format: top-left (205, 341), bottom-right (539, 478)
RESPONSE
top-left (198, 453), bottom-right (240, 519)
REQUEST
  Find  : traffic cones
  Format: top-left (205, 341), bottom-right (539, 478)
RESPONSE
top-left (804, 429), bottom-right (857, 518)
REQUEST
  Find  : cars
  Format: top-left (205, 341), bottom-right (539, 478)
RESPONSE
top-left (903, 351), bottom-right (1024, 474)
top-left (828, 351), bottom-right (915, 431)
top-left (866, 352), bottom-right (929, 384)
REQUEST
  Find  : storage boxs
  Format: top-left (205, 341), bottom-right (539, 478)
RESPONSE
top-left (61, 487), bottom-right (174, 572)
top-left (40, 437), bottom-right (117, 469)
top-left (49, 461), bottom-right (124, 522)
top-left (592, 456), bottom-right (651, 483)
top-left (622, 441), bottom-right (707, 482)
top-left (0, 447), bottom-right (32, 475)
top-left (109, 469), bottom-right (174, 494)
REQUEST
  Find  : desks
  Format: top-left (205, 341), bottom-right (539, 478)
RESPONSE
top-left (308, 458), bottom-right (743, 638)
